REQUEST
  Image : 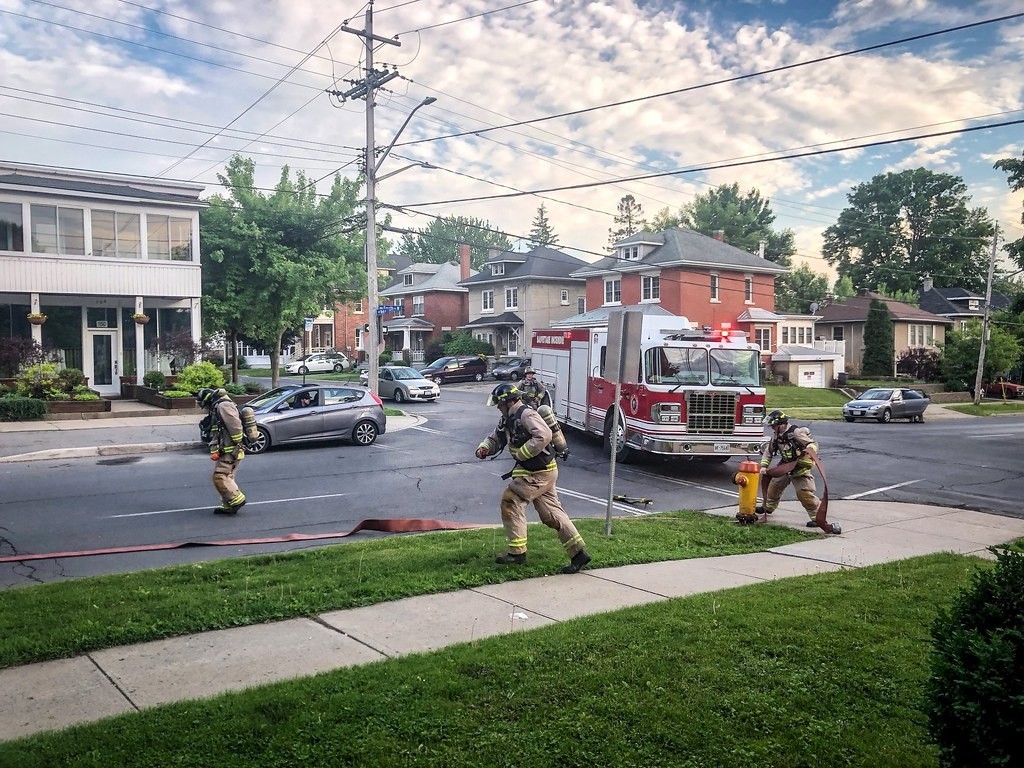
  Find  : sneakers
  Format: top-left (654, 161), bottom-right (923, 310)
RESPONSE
top-left (806, 521), bottom-right (818, 527)
top-left (755, 506), bottom-right (772, 514)
top-left (562, 548), bottom-right (592, 574)
top-left (495, 553), bottom-right (526, 565)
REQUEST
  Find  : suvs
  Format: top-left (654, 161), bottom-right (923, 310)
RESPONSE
top-left (418, 354), bottom-right (488, 385)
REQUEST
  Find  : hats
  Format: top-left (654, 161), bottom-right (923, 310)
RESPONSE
top-left (299, 392), bottom-right (313, 399)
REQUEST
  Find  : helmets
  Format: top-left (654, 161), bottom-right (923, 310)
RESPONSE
top-left (768, 410), bottom-right (790, 426)
top-left (524, 367), bottom-right (536, 374)
top-left (197, 388), bottom-right (215, 410)
top-left (492, 382), bottom-right (527, 406)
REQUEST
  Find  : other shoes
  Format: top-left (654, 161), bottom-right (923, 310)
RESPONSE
top-left (213, 507), bottom-right (229, 515)
top-left (227, 500), bottom-right (246, 515)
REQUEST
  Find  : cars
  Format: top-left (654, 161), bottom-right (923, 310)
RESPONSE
top-left (239, 381), bottom-right (387, 455)
top-left (285, 351), bottom-right (350, 376)
top-left (843, 387), bottom-right (930, 424)
top-left (490, 356), bottom-right (531, 381)
top-left (359, 366), bottom-right (441, 404)
top-left (981, 373), bottom-right (1024, 399)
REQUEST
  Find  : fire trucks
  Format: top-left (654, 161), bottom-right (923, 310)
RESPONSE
top-left (529, 315), bottom-right (768, 466)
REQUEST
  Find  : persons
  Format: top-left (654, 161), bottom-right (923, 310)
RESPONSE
top-left (284, 393), bottom-right (312, 408)
top-left (903, 390), bottom-right (924, 423)
top-left (981, 387), bottom-right (985, 398)
top-left (516, 366), bottom-right (546, 409)
top-left (756, 410), bottom-right (828, 526)
top-left (197, 388), bottom-right (246, 516)
top-left (475, 385), bottom-right (591, 573)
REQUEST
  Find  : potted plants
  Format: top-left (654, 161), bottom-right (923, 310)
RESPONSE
top-left (132, 314), bottom-right (150, 324)
top-left (122, 366), bottom-right (137, 399)
top-left (27, 313), bottom-right (47, 325)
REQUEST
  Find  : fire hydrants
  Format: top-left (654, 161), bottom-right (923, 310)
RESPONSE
top-left (732, 458), bottom-right (761, 525)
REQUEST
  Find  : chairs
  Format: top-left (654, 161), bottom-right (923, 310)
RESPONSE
top-left (314, 393), bottom-right (319, 405)
